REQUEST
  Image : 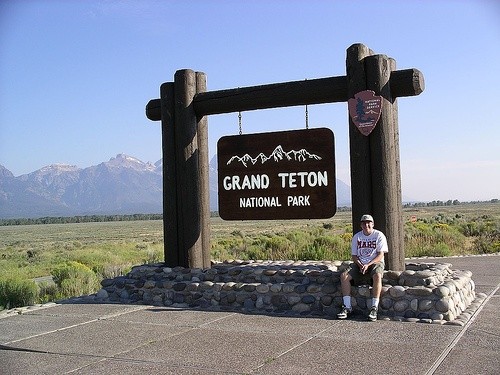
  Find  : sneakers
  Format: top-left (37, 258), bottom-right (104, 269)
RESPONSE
top-left (336, 305), bottom-right (352, 318)
top-left (368, 305), bottom-right (378, 321)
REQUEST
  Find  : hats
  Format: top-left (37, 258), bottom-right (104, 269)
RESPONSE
top-left (360, 215), bottom-right (373, 222)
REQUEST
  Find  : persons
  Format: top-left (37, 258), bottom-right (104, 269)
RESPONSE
top-left (337, 215), bottom-right (389, 321)
